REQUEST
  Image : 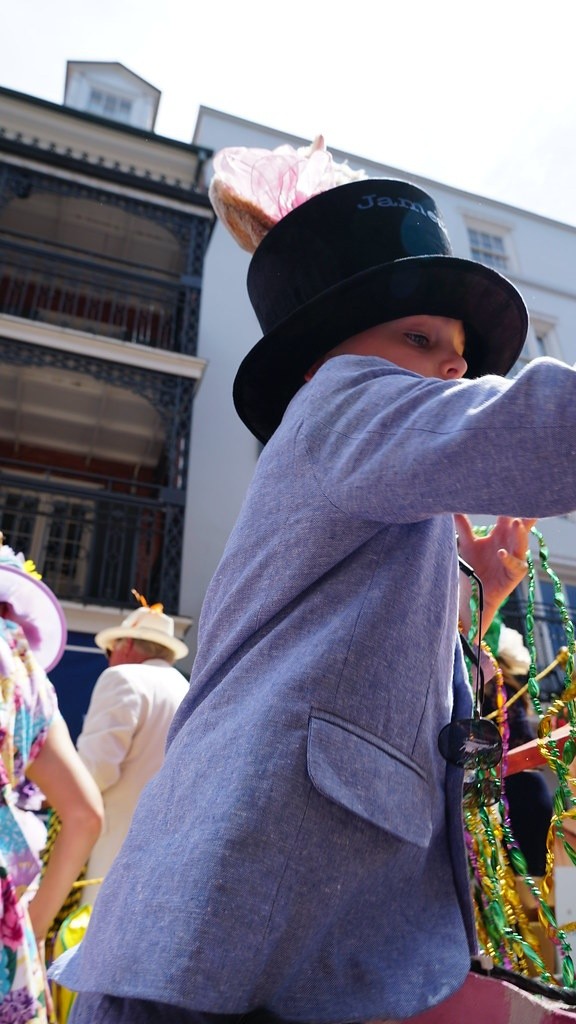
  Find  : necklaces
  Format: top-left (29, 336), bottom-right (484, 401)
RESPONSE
top-left (453, 523), bottom-right (576, 989)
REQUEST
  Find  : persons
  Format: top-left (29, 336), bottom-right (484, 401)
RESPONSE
top-left (77, 607), bottom-right (192, 910)
top-left (473, 631), bottom-right (554, 871)
top-left (47, 179), bottom-right (576, 1024)
top-left (0, 542), bottom-right (102, 1024)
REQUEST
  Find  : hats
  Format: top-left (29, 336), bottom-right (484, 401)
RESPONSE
top-left (94, 590), bottom-right (189, 660)
top-left (209, 134), bottom-right (530, 446)
top-left (0, 545), bottom-right (67, 674)
top-left (481, 623), bottom-right (532, 674)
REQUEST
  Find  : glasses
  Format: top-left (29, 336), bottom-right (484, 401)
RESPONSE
top-left (437, 556), bottom-right (504, 809)
top-left (105, 638), bottom-right (120, 659)
